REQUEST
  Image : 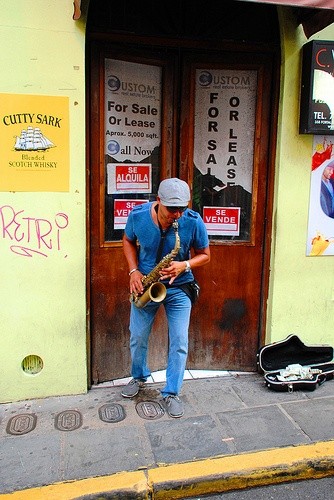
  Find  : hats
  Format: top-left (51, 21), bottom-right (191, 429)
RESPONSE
top-left (158, 177), bottom-right (190, 206)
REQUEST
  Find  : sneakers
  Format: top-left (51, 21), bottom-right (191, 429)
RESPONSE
top-left (121, 377), bottom-right (145, 397)
top-left (165, 394), bottom-right (184, 418)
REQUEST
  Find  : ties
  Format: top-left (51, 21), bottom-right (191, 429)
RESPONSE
top-left (154, 204), bottom-right (172, 264)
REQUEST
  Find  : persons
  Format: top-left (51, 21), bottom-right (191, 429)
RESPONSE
top-left (122, 177), bottom-right (210, 418)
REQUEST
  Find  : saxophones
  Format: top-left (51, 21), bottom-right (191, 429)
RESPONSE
top-left (129, 219), bottom-right (182, 310)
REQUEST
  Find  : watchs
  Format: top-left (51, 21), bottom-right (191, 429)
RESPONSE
top-left (184, 261), bottom-right (190, 274)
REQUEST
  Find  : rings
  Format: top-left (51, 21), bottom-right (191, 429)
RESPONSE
top-left (169, 274), bottom-right (172, 277)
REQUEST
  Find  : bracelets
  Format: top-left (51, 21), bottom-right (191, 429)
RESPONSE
top-left (128, 268), bottom-right (140, 276)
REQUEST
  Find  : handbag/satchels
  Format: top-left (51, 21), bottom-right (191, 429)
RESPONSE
top-left (179, 280), bottom-right (201, 305)
